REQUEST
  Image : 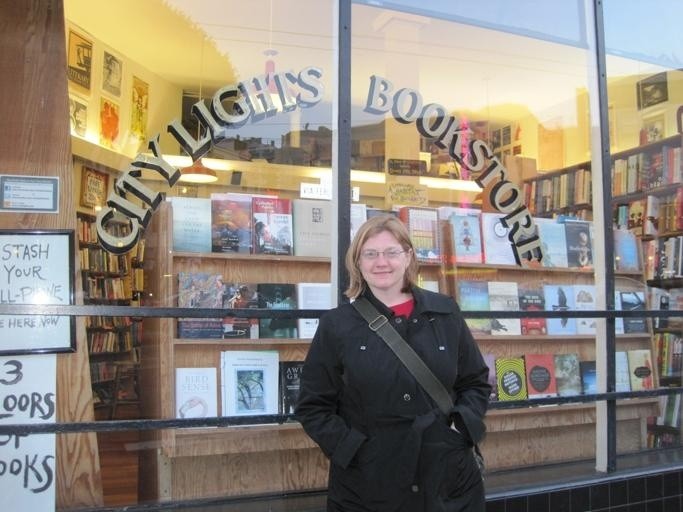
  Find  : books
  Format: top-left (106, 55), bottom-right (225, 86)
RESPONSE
top-left (75, 214), bottom-right (145, 408)
top-left (165, 137), bottom-right (682, 443)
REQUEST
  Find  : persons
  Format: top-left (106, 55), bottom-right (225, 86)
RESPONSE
top-left (293, 212), bottom-right (494, 512)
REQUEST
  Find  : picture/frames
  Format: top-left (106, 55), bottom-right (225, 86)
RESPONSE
top-left (0, 227), bottom-right (77, 358)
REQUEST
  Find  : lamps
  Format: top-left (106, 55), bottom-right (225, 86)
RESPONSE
top-left (175, 34), bottom-right (218, 184)
top-left (249, 0), bottom-right (297, 113)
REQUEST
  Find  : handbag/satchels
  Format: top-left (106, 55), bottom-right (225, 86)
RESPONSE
top-left (471, 440), bottom-right (486, 485)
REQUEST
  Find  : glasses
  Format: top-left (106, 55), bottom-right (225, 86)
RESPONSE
top-left (358, 246), bottom-right (407, 260)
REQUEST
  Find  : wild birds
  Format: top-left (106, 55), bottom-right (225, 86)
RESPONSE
top-left (552, 287), bottom-right (570, 328)
